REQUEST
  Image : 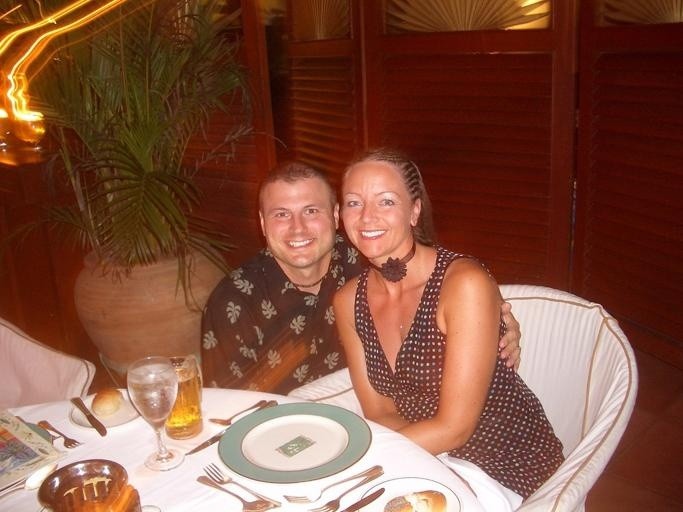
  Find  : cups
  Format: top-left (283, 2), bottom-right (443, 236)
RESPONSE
top-left (159, 355), bottom-right (203, 439)
top-left (25, 464), bottom-right (59, 512)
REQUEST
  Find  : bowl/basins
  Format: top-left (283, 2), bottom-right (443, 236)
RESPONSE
top-left (37, 459), bottom-right (128, 512)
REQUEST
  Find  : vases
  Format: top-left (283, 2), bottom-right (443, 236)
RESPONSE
top-left (24, 0), bottom-right (290, 383)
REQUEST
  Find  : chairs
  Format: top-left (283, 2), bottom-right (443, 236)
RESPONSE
top-left (285, 283), bottom-right (641, 512)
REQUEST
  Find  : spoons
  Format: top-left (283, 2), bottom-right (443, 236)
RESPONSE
top-left (197, 475), bottom-right (274, 512)
top-left (208, 401), bottom-right (268, 426)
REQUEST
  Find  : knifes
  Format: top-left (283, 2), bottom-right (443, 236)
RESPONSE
top-left (70, 396), bottom-right (107, 437)
top-left (183, 400), bottom-right (279, 456)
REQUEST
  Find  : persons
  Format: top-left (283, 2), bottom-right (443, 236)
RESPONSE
top-left (199, 162), bottom-right (522, 397)
top-left (331, 146), bottom-right (566, 503)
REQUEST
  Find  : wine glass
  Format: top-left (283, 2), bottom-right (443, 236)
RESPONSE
top-left (127, 355), bottom-right (185, 471)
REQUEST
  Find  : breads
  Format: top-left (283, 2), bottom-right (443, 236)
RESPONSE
top-left (92, 388), bottom-right (123, 415)
top-left (382, 489), bottom-right (448, 512)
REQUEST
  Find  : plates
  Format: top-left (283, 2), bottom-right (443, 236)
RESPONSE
top-left (218, 402), bottom-right (371, 483)
top-left (359, 477), bottom-right (461, 512)
top-left (69, 389), bottom-right (140, 429)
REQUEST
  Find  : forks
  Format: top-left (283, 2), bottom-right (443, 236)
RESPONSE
top-left (203, 463), bottom-right (284, 507)
top-left (39, 420), bottom-right (84, 449)
top-left (282, 465), bottom-right (384, 512)
